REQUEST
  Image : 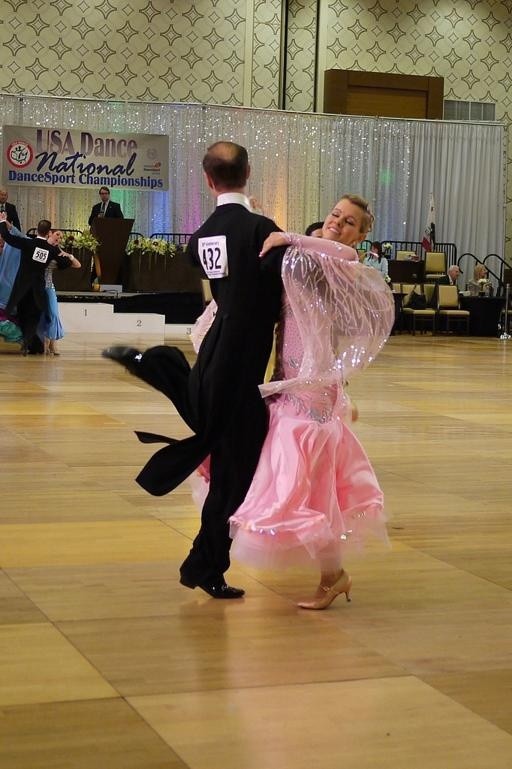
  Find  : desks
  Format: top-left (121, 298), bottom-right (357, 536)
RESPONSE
top-left (462, 295), bottom-right (506, 336)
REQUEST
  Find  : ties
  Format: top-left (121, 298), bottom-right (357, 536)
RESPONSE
top-left (101, 203), bottom-right (105, 219)
top-left (0, 203), bottom-right (5, 212)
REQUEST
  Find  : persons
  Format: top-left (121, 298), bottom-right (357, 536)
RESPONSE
top-left (0, 219), bottom-right (14, 256)
top-left (0, 189), bottom-right (22, 233)
top-left (357, 240), bottom-right (389, 337)
top-left (99, 140), bottom-right (355, 601)
top-left (302, 220), bottom-right (326, 238)
top-left (87, 186), bottom-right (125, 285)
top-left (430, 264), bottom-right (462, 335)
top-left (466, 263), bottom-right (492, 297)
top-left (187, 189), bottom-right (395, 611)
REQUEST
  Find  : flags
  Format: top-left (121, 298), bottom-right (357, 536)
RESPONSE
top-left (420, 191), bottom-right (436, 253)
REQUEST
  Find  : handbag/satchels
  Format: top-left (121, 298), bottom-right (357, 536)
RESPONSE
top-left (411, 294), bottom-right (426, 310)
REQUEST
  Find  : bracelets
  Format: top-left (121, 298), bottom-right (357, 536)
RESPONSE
top-left (69, 253), bottom-right (75, 261)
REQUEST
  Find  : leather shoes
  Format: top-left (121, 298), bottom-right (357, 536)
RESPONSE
top-left (178, 571), bottom-right (244, 599)
top-left (102, 345), bottom-right (143, 376)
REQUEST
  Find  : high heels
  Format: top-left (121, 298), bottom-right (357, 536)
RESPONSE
top-left (296, 567), bottom-right (351, 610)
top-left (46, 344), bottom-right (60, 355)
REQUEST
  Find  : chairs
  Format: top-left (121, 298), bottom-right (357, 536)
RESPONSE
top-left (385, 247), bottom-right (471, 339)
